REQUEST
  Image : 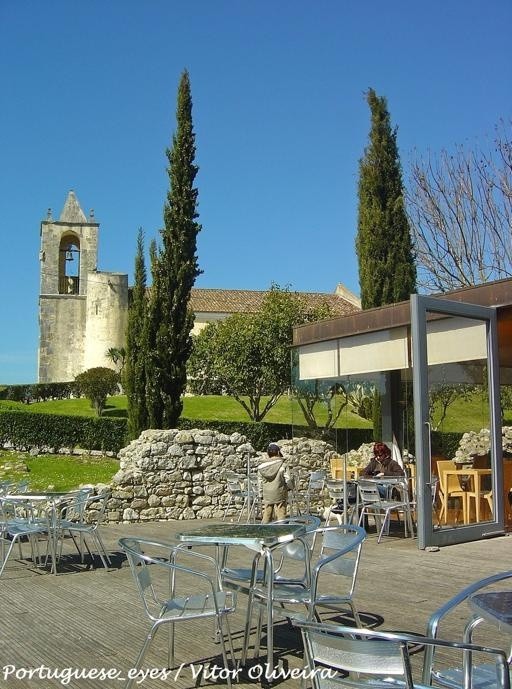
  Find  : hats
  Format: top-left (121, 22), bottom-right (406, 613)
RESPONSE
top-left (268, 445), bottom-right (282, 458)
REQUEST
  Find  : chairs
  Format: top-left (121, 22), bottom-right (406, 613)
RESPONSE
top-left (321, 454), bottom-right (512, 545)
top-left (222, 466), bottom-right (328, 526)
top-left (118, 513), bottom-right (512, 689)
top-left (0, 483), bottom-right (113, 575)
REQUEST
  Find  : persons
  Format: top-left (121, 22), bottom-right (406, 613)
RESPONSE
top-left (329, 441), bottom-right (406, 532)
top-left (256, 442), bottom-right (296, 544)
